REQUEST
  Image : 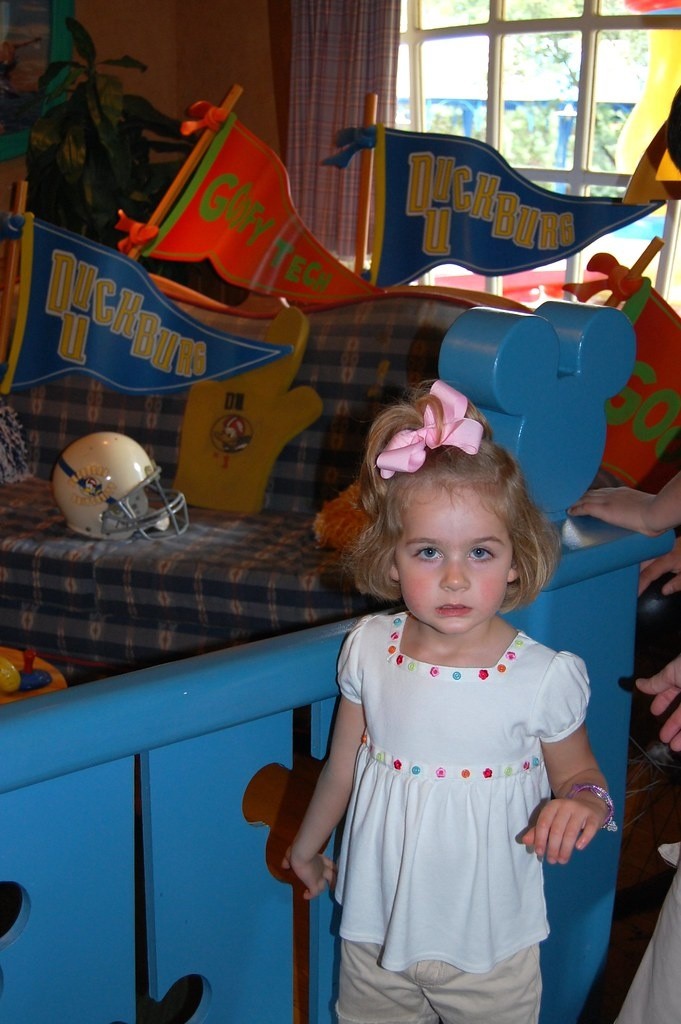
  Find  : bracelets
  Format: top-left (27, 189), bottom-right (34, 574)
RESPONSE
top-left (567, 783), bottom-right (617, 832)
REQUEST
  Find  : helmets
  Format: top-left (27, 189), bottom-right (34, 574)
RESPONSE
top-left (52, 431), bottom-right (170, 542)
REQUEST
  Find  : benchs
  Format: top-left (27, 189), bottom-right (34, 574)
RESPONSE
top-left (0, 273), bottom-right (534, 687)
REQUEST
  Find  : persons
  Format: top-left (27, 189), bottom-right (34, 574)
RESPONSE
top-left (281, 379), bottom-right (610, 1024)
top-left (566, 85), bottom-right (680, 1024)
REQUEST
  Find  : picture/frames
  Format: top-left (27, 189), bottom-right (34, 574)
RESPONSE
top-left (0, 0), bottom-right (75, 163)
